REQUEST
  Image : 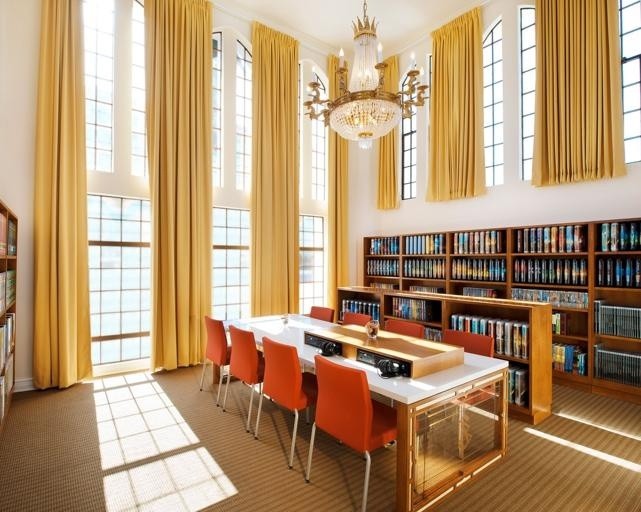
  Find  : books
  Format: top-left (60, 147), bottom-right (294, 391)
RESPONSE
top-left (463, 287), bottom-right (500, 298)
top-left (452, 313), bottom-right (529, 360)
top-left (602, 224), bottom-right (639, 252)
top-left (452, 258), bottom-right (506, 282)
top-left (552, 310), bottom-right (571, 336)
top-left (367, 259), bottom-right (400, 277)
top-left (392, 297), bottom-right (433, 321)
top-left (454, 231), bottom-right (506, 254)
top-left (512, 288), bottom-right (588, 308)
top-left (605, 306), bottom-right (639, 338)
top-left (406, 234), bottom-right (446, 255)
top-left (515, 258), bottom-right (587, 285)
top-left (508, 366), bottom-right (527, 405)
top-left (552, 342), bottom-right (588, 376)
top-left (604, 349), bottom-right (640, 388)
top-left (409, 286), bottom-right (444, 293)
top-left (594, 300), bottom-right (606, 334)
top-left (518, 226), bottom-right (587, 253)
top-left (1, 214), bottom-right (17, 420)
top-left (404, 259), bottom-right (446, 279)
top-left (592, 343), bottom-right (604, 378)
top-left (370, 237), bottom-right (399, 255)
top-left (599, 257), bottom-right (639, 287)
top-left (425, 327), bottom-right (442, 343)
top-left (370, 282), bottom-right (399, 290)
top-left (342, 299), bottom-right (380, 324)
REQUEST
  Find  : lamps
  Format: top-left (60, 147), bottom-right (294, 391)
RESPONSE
top-left (302, 0), bottom-right (430, 149)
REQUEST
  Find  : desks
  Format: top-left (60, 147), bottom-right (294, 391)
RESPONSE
top-left (212, 314), bottom-right (509, 512)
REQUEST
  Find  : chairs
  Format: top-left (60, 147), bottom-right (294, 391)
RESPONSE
top-left (199, 306), bottom-right (419, 512)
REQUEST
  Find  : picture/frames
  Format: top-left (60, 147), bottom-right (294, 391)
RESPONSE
top-left (212, 314), bottom-right (509, 512)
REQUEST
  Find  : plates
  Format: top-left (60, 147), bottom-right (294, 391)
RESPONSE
top-left (0, 199), bottom-right (18, 434)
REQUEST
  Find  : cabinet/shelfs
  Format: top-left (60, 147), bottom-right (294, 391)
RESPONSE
top-left (0, 199), bottom-right (18, 434)
top-left (337, 217), bottom-right (641, 426)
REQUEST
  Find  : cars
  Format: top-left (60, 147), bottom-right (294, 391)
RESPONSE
top-left (366, 320), bottom-right (379, 339)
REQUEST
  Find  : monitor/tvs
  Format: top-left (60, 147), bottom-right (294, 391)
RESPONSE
top-left (1, 214), bottom-right (17, 420)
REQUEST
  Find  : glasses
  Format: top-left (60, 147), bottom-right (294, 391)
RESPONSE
top-left (302, 0), bottom-right (430, 149)
